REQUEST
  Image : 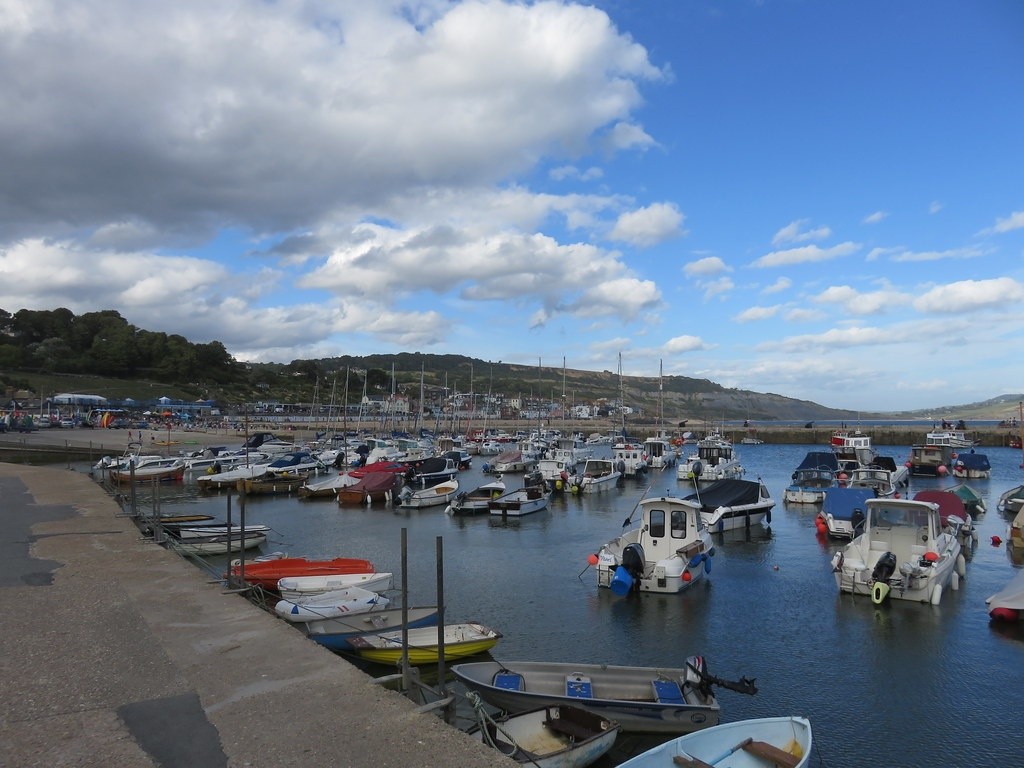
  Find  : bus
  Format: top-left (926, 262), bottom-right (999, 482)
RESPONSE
top-left (82, 408), bottom-right (133, 427)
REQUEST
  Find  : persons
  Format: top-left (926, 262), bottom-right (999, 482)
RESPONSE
top-left (139, 431), bottom-right (141, 439)
top-left (405, 462), bottom-right (413, 484)
top-left (151, 433), bottom-right (154, 440)
top-left (214, 461), bottom-right (221, 474)
top-left (128, 431), bottom-right (133, 441)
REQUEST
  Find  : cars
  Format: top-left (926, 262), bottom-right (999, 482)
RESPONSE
top-left (0, 412), bottom-right (76, 432)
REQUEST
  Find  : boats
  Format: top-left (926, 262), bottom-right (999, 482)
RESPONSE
top-left (1008, 440), bottom-right (1021, 449)
top-left (828, 496), bottom-right (968, 608)
top-left (815, 486), bottom-right (879, 541)
top-left (450, 653), bottom-right (761, 735)
top-left (1004, 503), bottom-right (1024, 567)
top-left (274, 585), bottom-right (390, 624)
top-left (226, 556), bottom-right (375, 591)
top-left (343, 619), bottom-right (505, 669)
top-left (179, 523), bottom-right (271, 539)
top-left (303, 606), bottom-right (447, 651)
top-left (174, 531), bottom-right (268, 556)
top-left (952, 450), bottom-right (991, 479)
top-left (943, 482), bottom-right (983, 515)
top-left (681, 476), bottom-right (776, 535)
top-left (466, 701), bottom-right (621, 768)
top-left (925, 427), bottom-right (982, 450)
top-left (898, 489), bottom-right (978, 548)
top-left (984, 568), bottom-right (1024, 625)
top-left (277, 572), bottom-right (395, 601)
top-left (781, 423), bottom-right (910, 505)
top-left (907, 442), bottom-right (955, 476)
top-left (578, 461), bottom-right (716, 594)
top-left (614, 714), bottom-right (813, 768)
top-left (86, 350), bottom-right (767, 534)
top-left (997, 484), bottom-right (1024, 514)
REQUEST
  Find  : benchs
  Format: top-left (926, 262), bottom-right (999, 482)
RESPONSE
top-left (387, 637), bottom-right (404, 648)
top-left (544, 717), bottom-right (597, 740)
top-left (457, 627), bottom-right (488, 640)
top-left (651, 680), bottom-right (687, 705)
top-left (284, 583), bottom-right (298, 587)
top-left (326, 580), bottom-right (341, 586)
top-left (335, 601), bottom-right (349, 612)
top-left (673, 753), bottom-right (715, 768)
top-left (565, 675), bottom-right (593, 698)
top-left (742, 741), bottom-right (802, 768)
top-left (494, 673), bottom-right (523, 691)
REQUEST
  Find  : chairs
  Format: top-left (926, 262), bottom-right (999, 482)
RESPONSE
top-left (911, 544), bottom-right (926, 564)
top-left (868, 541), bottom-right (887, 566)
